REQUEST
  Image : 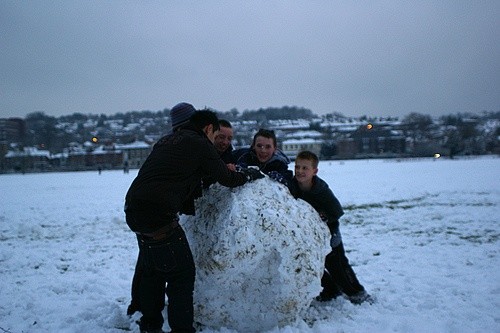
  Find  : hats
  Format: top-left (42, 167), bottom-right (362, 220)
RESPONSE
top-left (169, 102), bottom-right (197, 129)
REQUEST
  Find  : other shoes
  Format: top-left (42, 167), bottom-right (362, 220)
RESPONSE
top-left (316, 287), bottom-right (341, 302)
top-left (351, 294), bottom-right (375, 305)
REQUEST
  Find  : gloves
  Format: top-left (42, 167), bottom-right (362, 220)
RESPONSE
top-left (330, 230), bottom-right (342, 248)
top-left (268, 171), bottom-right (288, 186)
top-left (243, 167), bottom-right (265, 183)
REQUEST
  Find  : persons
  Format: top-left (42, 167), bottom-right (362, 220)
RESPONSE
top-left (288, 151), bottom-right (373, 307)
top-left (124, 102), bottom-right (293, 333)
